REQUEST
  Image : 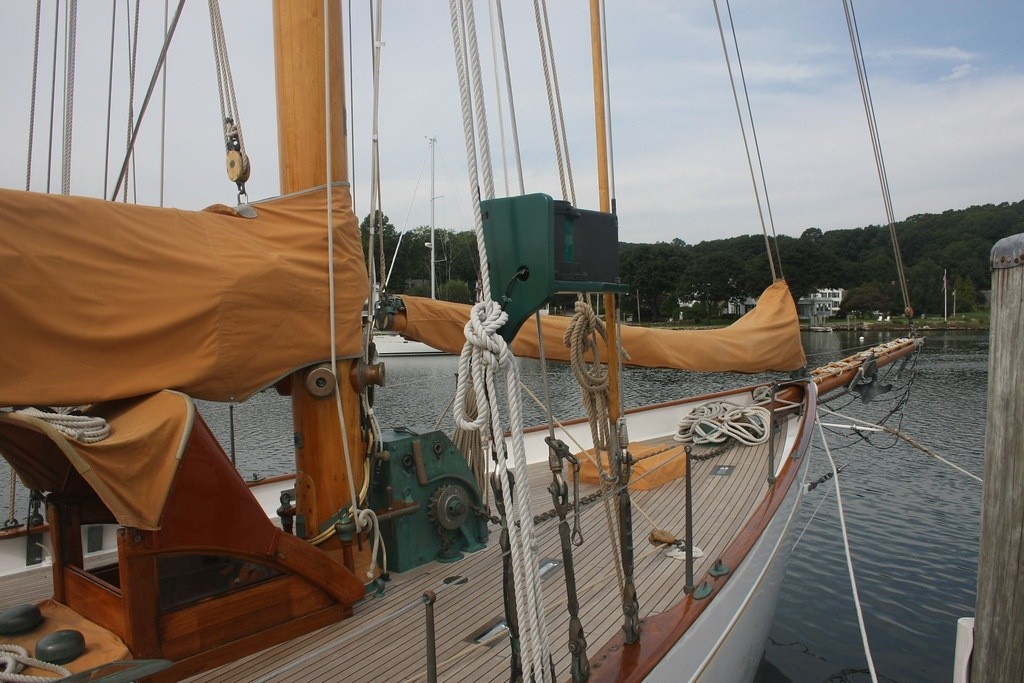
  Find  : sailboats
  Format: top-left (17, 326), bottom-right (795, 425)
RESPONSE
top-left (0, 2), bottom-right (928, 683)
